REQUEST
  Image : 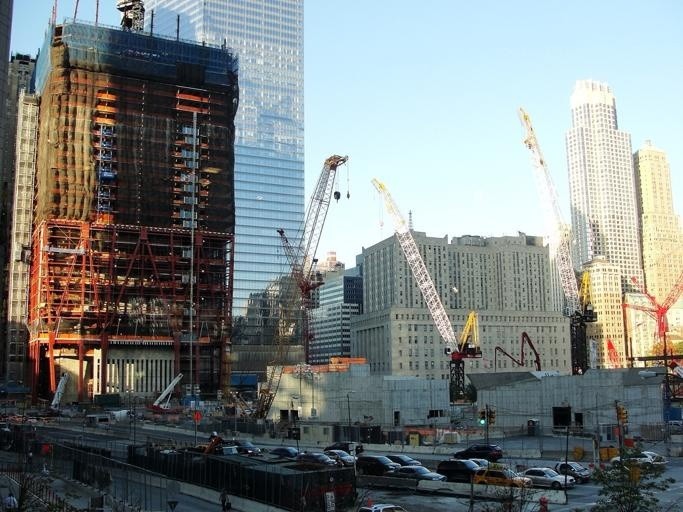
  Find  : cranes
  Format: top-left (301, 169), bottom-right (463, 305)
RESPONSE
top-left (621, 272), bottom-right (683, 371)
top-left (521, 109), bottom-right (599, 375)
top-left (223, 155), bottom-right (352, 426)
top-left (371, 176), bottom-right (483, 399)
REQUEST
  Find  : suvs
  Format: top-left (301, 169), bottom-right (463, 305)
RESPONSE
top-left (437, 459), bottom-right (479, 481)
top-left (356, 456), bottom-right (399, 475)
top-left (454, 441), bottom-right (502, 462)
top-left (475, 464), bottom-right (532, 489)
top-left (325, 441), bottom-right (363, 453)
top-left (389, 454), bottom-right (421, 466)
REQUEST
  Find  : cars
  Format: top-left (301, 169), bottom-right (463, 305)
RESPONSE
top-left (396, 466), bottom-right (445, 481)
top-left (272, 449), bottom-right (355, 468)
top-left (611, 450), bottom-right (667, 470)
top-left (224, 439), bottom-right (261, 454)
top-left (517, 467), bottom-right (575, 488)
top-left (557, 462), bottom-right (591, 484)
top-left (469, 457), bottom-right (490, 466)
top-left (359, 503), bottom-right (405, 511)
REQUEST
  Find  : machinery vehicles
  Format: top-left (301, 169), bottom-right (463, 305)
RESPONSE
top-left (112, 410), bottom-right (134, 424)
top-left (151, 373), bottom-right (183, 414)
top-left (49, 370), bottom-right (79, 418)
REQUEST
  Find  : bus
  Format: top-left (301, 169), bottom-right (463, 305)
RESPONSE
top-left (85, 412), bottom-right (110, 428)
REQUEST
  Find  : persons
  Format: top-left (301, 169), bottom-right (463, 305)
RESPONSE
top-left (217, 486), bottom-right (228, 512)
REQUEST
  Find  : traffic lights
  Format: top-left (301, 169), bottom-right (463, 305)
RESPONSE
top-left (479, 410), bottom-right (486, 425)
top-left (618, 404), bottom-right (628, 423)
top-left (489, 408), bottom-right (496, 424)
